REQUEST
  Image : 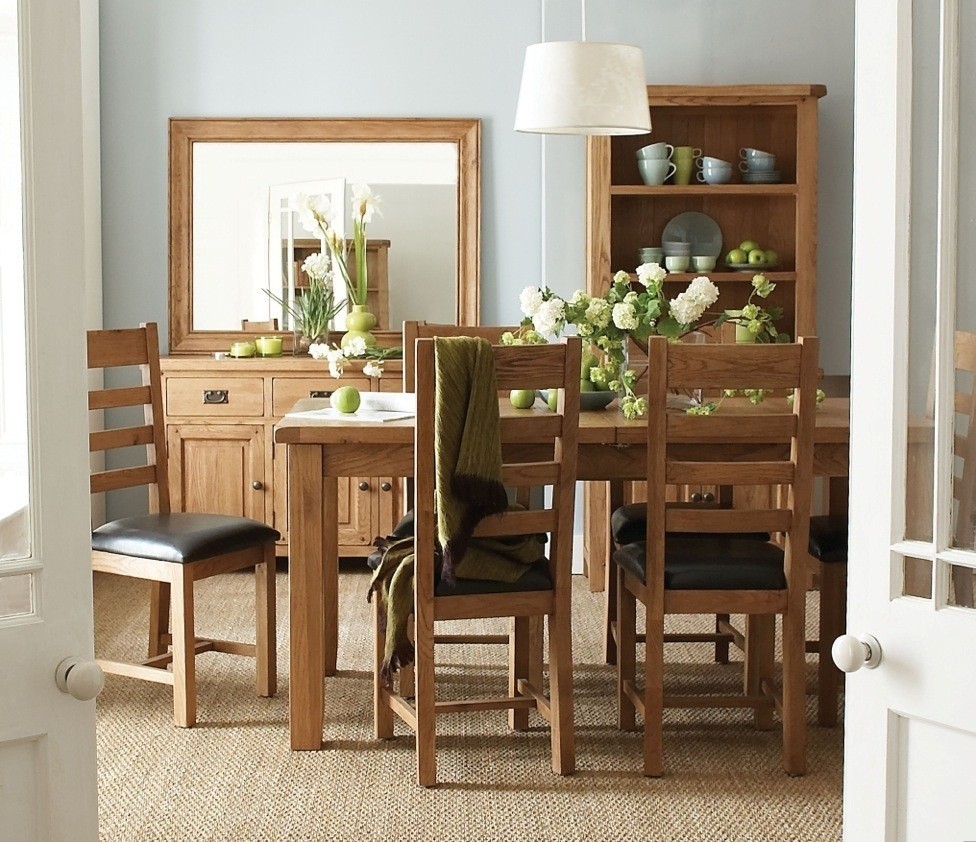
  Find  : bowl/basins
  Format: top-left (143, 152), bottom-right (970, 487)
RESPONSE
top-left (638, 241), bottom-right (716, 274)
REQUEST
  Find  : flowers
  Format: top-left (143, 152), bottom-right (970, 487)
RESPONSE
top-left (308, 340), bottom-right (404, 379)
top-left (499, 262), bottom-right (824, 419)
top-left (291, 183), bottom-right (380, 305)
top-left (262, 251), bottom-right (349, 354)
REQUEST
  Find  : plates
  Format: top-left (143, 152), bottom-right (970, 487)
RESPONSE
top-left (743, 171), bottom-right (782, 182)
top-left (662, 212), bottom-right (723, 259)
top-left (541, 389), bottom-right (616, 407)
top-left (723, 261), bottom-right (778, 272)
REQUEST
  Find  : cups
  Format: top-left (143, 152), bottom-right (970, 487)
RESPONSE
top-left (695, 155), bottom-right (733, 185)
top-left (738, 147), bottom-right (775, 172)
top-left (256, 334), bottom-right (283, 358)
top-left (230, 342), bottom-right (256, 357)
top-left (725, 308), bottom-right (756, 344)
top-left (669, 146), bottom-right (701, 184)
top-left (636, 142), bottom-right (676, 185)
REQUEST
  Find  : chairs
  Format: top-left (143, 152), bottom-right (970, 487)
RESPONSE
top-left (60, 321), bottom-right (976, 786)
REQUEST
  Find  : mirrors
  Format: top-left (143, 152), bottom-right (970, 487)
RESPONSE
top-left (166, 115), bottom-right (481, 354)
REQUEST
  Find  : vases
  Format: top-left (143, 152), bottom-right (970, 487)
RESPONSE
top-left (293, 319), bottom-right (329, 357)
top-left (341, 304), bottom-right (376, 356)
top-left (648, 331), bottom-right (705, 414)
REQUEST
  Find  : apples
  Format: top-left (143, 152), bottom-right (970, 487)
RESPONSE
top-left (726, 240), bottom-right (779, 264)
top-left (547, 389), bottom-right (558, 412)
top-left (581, 353), bottom-right (619, 392)
top-left (510, 389), bottom-right (536, 409)
top-left (329, 386), bottom-right (361, 413)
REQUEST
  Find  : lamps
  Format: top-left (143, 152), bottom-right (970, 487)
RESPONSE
top-left (508, 0), bottom-right (654, 138)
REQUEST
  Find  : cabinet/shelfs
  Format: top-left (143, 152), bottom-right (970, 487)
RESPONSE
top-left (588, 83), bottom-right (826, 405)
top-left (149, 363), bottom-right (266, 554)
top-left (279, 238), bottom-right (392, 331)
top-left (270, 361), bottom-right (436, 557)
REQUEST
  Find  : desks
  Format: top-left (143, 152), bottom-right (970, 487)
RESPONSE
top-left (273, 395), bottom-right (935, 750)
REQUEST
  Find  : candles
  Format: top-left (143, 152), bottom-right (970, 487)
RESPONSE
top-left (255, 339), bottom-right (282, 354)
top-left (230, 341), bottom-right (254, 356)
